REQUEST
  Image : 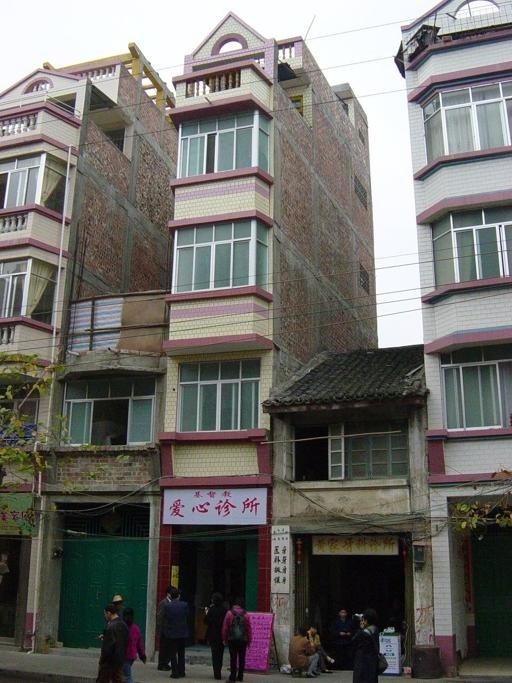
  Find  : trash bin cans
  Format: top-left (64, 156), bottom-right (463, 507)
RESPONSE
top-left (411, 644), bottom-right (442, 679)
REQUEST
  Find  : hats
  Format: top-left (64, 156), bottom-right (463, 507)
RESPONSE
top-left (110, 595), bottom-right (123, 604)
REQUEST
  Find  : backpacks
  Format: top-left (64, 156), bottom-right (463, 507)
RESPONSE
top-left (229, 609), bottom-right (249, 646)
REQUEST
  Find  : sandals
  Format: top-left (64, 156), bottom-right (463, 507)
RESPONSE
top-left (306, 657), bottom-right (336, 677)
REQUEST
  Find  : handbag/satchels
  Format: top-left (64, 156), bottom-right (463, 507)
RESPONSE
top-left (377, 653), bottom-right (388, 675)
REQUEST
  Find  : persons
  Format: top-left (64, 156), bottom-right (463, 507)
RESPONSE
top-left (94, 603), bottom-right (129, 682)
top-left (306, 622), bottom-right (336, 673)
top-left (121, 608), bottom-right (147, 683)
top-left (289, 626), bottom-right (320, 678)
top-left (333, 606), bottom-right (387, 683)
top-left (159, 586), bottom-right (192, 678)
top-left (221, 598), bottom-right (253, 682)
top-left (204, 593), bottom-right (226, 680)
top-left (330, 608), bottom-right (355, 668)
top-left (156, 586), bottom-right (177, 672)
top-left (112, 594), bottom-right (126, 621)
